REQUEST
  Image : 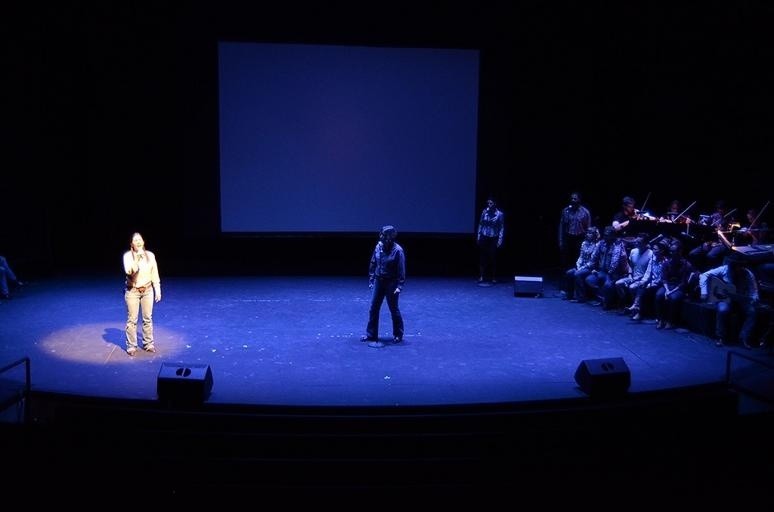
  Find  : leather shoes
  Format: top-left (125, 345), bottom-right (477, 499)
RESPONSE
top-left (128, 348), bottom-right (155, 356)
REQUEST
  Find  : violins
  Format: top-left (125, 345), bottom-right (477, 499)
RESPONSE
top-left (676, 212), bottom-right (696, 224)
top-left (629, 208), bottom-right (650, 220)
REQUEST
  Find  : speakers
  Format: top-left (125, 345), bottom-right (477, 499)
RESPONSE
top-left (513, 276), bottom-right (543, 297)
top-left (156, 363), bottom-right (214, 401)
top-left (573, 357), bottom-right (631, 394)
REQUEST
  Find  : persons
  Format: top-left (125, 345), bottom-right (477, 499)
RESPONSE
top-left (0, 256), bottom-right (31, 300)
top-left (558, 193), bottom-right (774, 350)
top-left (123, 232), bottom-right (162, 356)
top-left (477, 199), bottom-right (505, 284)
top-left (360, 225), bottom-right (405, 344)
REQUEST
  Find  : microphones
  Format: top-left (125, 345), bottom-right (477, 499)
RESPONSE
top-left (138, 247), bottom-right (144, 258)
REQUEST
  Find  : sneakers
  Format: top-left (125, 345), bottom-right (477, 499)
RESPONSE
top-left (625, 303), bottom-right (640, 312)
top-left (594, 303), bottom-right (601, 307)
top-left (633, 313), bottom-right (641, 320)
top-left (718, 339), bottom-right (726, 346)
top-left (658, 320), bottom-right (672, 328)
top-left (362, 335), bottom-right (402, 344)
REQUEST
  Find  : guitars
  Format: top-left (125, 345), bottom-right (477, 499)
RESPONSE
top-left (709, 275), bottom-right (768, 311)
